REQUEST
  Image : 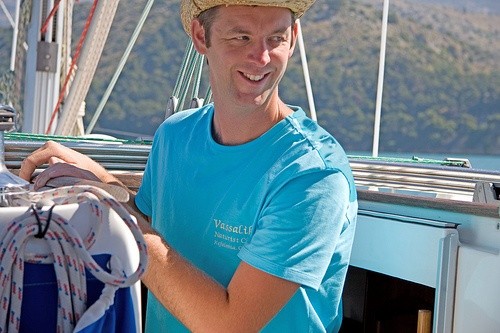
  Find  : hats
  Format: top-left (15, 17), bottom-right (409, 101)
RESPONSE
top-left (180, 0), bottom-right (316, 37)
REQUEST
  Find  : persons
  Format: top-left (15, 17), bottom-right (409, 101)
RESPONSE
top-left (19, 0), bottom-right (359, 333)
